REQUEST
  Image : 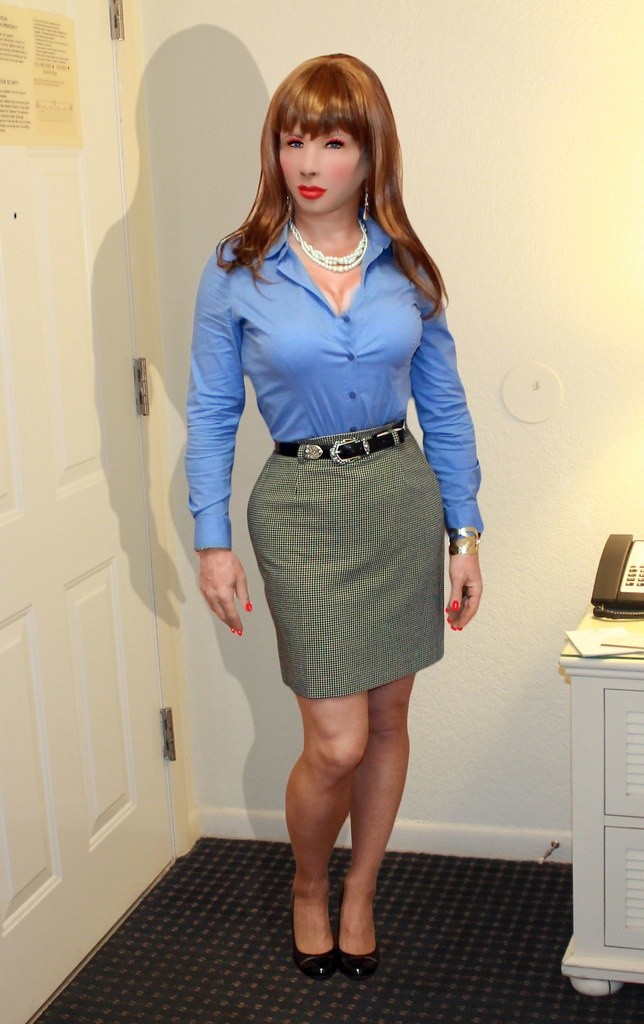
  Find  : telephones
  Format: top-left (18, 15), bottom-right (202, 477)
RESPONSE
top-left (591, 534), bottom-right (644, 620)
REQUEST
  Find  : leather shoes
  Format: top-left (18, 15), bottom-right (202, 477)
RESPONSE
top-left (338, 886), bottom-right (379, 981)
top-left (289, 889), bottom-right (337, 981)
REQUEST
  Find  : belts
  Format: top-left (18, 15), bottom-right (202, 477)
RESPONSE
top-left (272, 420), bottom-right (421, 466)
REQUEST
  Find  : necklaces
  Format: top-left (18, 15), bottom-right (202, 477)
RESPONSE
top-left (289, 213), bottom-right (369, 270)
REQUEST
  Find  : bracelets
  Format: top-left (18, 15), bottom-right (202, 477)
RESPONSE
top-left (449, 526), bottom-right (479, 556)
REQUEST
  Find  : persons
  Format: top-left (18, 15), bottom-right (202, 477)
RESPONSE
top-left (186, 54), bottom-right (484, 980)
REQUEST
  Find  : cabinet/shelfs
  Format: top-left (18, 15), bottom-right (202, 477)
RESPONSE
top-left (557, 603), bottom-right (644, 996)
